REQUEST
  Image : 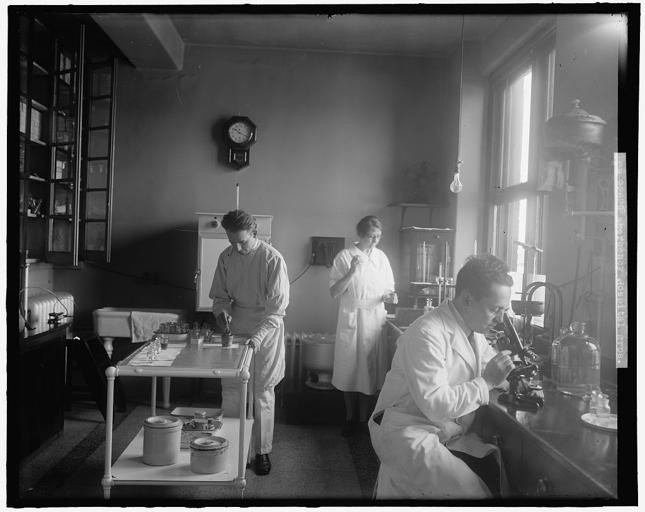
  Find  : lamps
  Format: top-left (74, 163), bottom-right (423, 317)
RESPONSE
top-left (541, 98), bottom-right (614, 243)
top-left (448, 14), bottom-right (465, 193)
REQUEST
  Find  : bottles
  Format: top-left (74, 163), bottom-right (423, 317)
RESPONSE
top-left (551, 322), bottom-right (602, 396)
top-left (589, 390), bottom-right (610, 425)
top-left (193, 409), bottom-right (208, 429)
top-left (146, 338), bottom-right (161, 361)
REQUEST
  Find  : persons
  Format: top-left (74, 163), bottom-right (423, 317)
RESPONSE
top-left (208, 210), bottom-right (292, 475)
top-left (326, 215), bottom-right (399, 445)
top-left (368, 253), bottom-right (538, 499)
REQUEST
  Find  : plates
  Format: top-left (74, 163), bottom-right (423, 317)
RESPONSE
top-left (581, 412), bottom-right (618, 434)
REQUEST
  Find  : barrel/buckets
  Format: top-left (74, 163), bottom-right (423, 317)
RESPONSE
top-left (143, 415), bottom-right (182, 466)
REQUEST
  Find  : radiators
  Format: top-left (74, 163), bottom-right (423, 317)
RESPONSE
top-left (27, 288), bottom-right (74, 335)
top-left (275, 330), bottom-right (334, 400)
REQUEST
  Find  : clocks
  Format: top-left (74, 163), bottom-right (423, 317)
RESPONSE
top-left (224, 110), bottom-right (257, 172)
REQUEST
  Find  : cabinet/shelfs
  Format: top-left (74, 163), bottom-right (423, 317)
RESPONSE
top-left (17, 6), bottom-right (118, 270)
top-left (387, 201), bottom-right (457, 310)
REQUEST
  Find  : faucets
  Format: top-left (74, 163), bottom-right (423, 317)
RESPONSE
top-left (523, 281), bottom-right (563, 341)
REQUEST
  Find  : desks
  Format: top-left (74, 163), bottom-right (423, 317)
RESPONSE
top-left (101, 333), bottom-right (254, 499)
top-left (384, 317), bottom-right (618, 500)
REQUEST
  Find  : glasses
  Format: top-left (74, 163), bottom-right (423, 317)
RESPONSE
top-left (365, 232), bottom-right (381, 239)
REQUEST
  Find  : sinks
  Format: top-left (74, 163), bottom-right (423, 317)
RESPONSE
top-left (95, 306), bottom-right (185, 338)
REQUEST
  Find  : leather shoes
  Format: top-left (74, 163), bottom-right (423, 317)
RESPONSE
top-left (254, 454), bottom-right (271, 475)
top-left (360, 420), bottom-right (370, 433)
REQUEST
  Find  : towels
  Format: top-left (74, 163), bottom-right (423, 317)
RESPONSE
top-left (130, 311), bottom-right (179, 344)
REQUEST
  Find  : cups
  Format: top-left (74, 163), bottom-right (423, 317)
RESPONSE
top-left (222, 335), bottom-right (233, 346)
top-left (160, 337), bottom-right (168, 350)
